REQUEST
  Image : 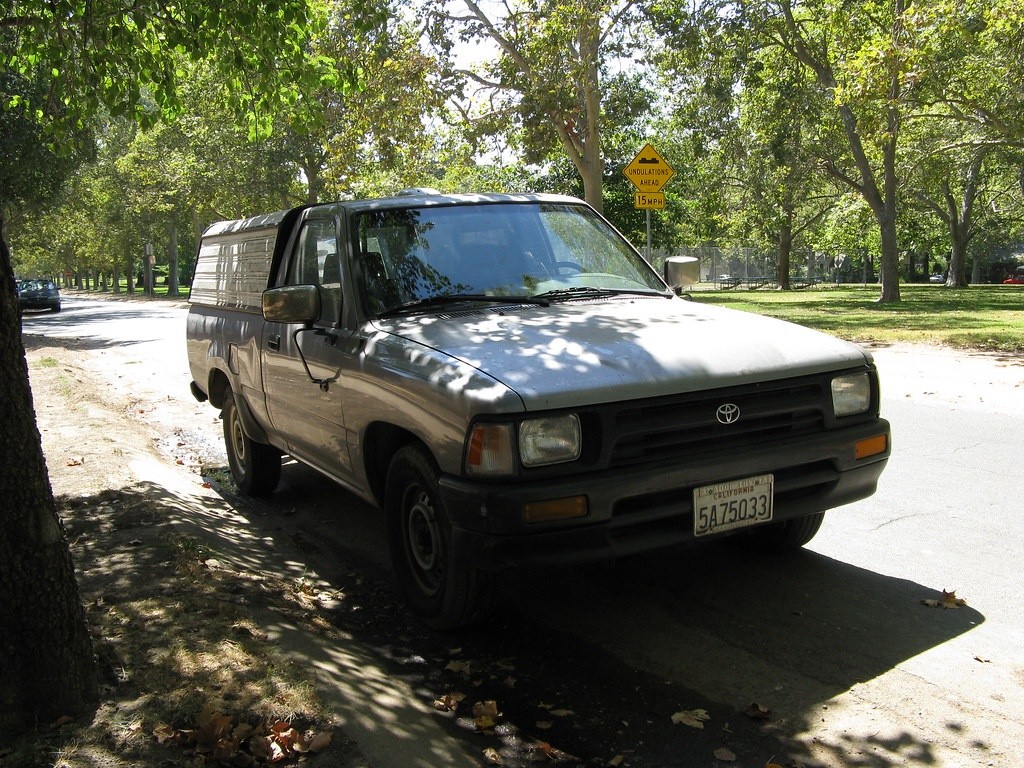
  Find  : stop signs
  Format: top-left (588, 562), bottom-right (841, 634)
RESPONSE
top-left (66, 271), bottom-right (71, 277)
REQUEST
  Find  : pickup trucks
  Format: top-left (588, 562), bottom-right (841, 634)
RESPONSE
top-left (185, 190), bottom-right (892, 633)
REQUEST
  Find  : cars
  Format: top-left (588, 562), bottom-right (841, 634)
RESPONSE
top-left (18, 280), bottom-right (62, 313)
top-left (1004, 275), bottom-right (1024, 283)
top-left (718, 273), bottom-right (733, 281)
top-left (929, 275), bottom-right (947, 283)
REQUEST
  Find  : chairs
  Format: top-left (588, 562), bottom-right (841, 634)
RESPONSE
top-left (323, 252), bottom-right (387, 285)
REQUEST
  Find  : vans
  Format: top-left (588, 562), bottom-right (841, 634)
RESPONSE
top-left (1017, 266), bottom-right (1024, 274)
